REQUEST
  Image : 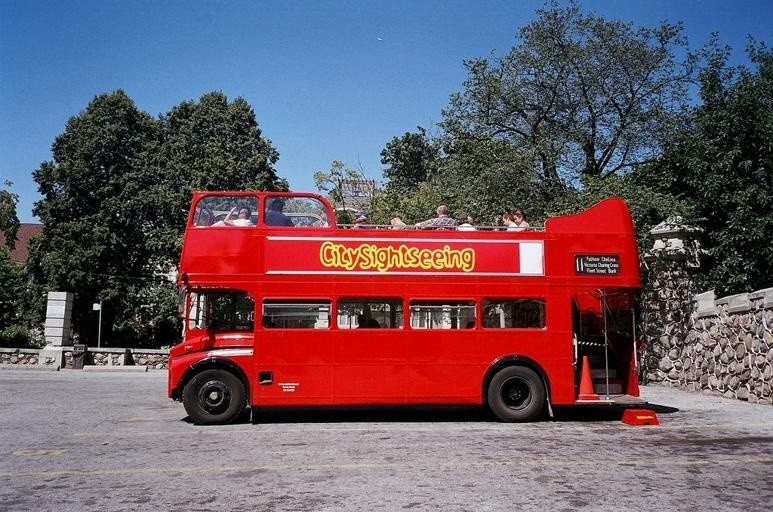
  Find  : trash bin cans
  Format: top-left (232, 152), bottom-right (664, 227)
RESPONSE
top-left (71, 344), bottom-right (88, 369)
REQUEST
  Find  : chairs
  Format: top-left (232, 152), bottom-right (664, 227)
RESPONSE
top-left (462, 321), bottom-right (474, 328)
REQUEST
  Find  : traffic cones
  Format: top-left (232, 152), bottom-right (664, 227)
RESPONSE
top-left (575, 355), bottom-right (600, 401)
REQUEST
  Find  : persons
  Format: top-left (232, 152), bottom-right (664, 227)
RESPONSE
top-left (354, 211), bottom-right (371, 229)
top-left (513, 210), bottom-right (530, 231)
top-left (455, 214), bottom-right (476, 230)
top-left (264, 200), bottom-right (294, 226)
top-left (211, 206), bottom-right (255, 226)
top-left (357, 315), bottom-right (364, 328)
top-left (390, 213), bottom-right (406, 230)
top-left (503, 214), bottom-right (518, 232)
top-left (414, 205), bottom-right (459, 231)
top-left (368, 319), bottom-right (380, 328)
top-left (321, 208), bottom-right (329, 227)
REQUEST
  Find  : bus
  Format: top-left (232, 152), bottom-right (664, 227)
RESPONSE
top-left (166, 189), bottom-right (645, 424)
